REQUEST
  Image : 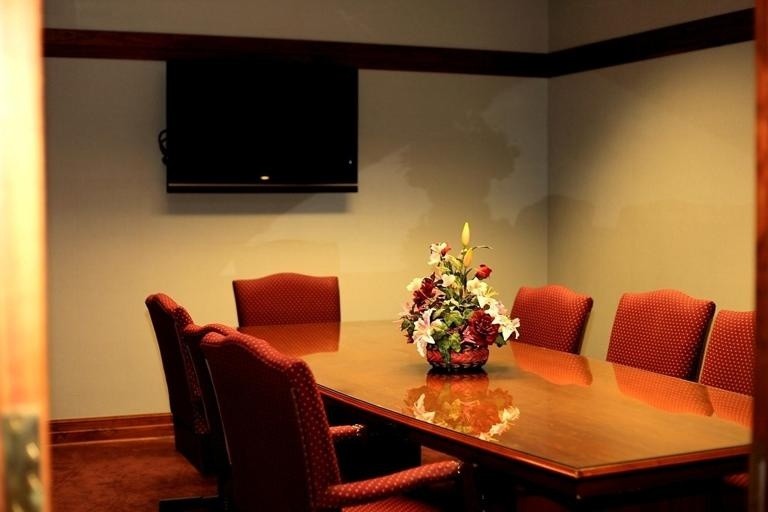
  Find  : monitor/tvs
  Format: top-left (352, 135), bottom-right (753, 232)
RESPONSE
top-left (162, 31), bottom-right (360, 194)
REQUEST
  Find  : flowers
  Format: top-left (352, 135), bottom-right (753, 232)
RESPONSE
top-left (400, 219), bottom-right (522, 350)
